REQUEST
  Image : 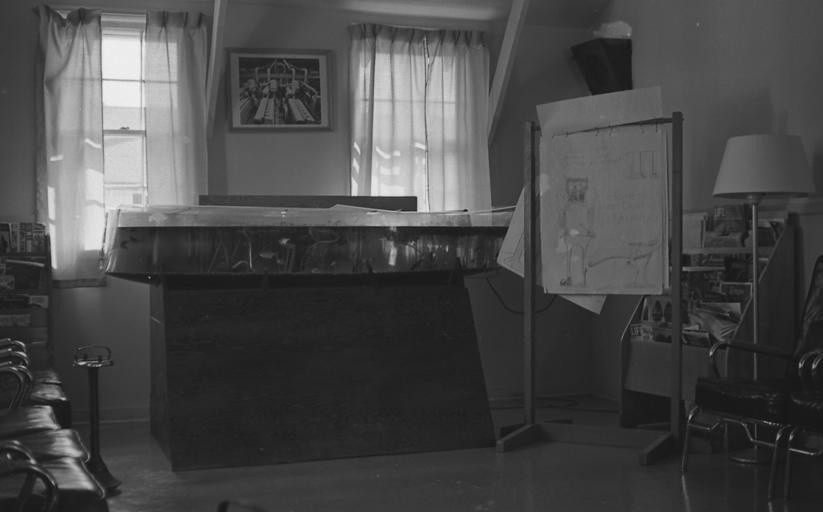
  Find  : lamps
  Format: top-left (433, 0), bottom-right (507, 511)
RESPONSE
top-left (709, 127), bottom-right (817, 468)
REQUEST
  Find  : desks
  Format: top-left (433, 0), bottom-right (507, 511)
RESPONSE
top-left (148, 271), bottom-right (495, 473)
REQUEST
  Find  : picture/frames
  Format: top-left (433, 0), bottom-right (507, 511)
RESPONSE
top-left (224, 44), bottom-right (338, 135)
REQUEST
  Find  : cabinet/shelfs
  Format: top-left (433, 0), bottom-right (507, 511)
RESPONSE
top-left (613, 206), bottom-right (800, 459)
top-left (0, 234), bottom-right (56, 371)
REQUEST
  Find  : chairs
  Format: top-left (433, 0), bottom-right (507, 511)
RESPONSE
top-left (0, 335), bottom-right (111, 512)
top-left (678, 254), bottom-right (822, 504)
top-left (775, 352), bottom-right (822, 503)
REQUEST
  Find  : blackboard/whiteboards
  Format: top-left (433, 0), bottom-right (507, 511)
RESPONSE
top-left (534, 118), bottom-right (673, 296)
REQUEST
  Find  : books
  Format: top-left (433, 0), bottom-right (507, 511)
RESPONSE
top-left (629, 203), bottom-right (788, 348)
